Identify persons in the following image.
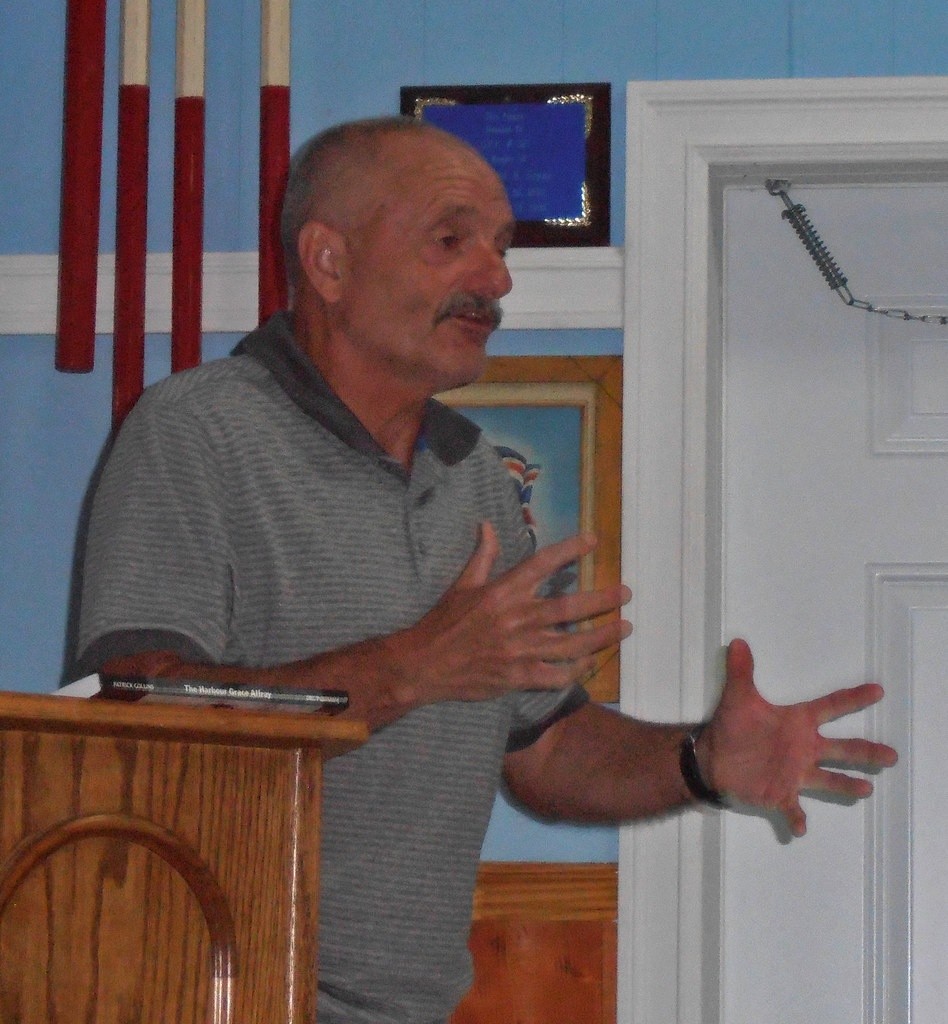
[71,115,900,1024]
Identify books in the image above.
[49,671,349,715]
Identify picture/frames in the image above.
[399,83,613,248]
[430,354,622,703]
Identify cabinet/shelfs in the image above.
[0,693,369,1024]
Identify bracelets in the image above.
[680,721,727,810]
